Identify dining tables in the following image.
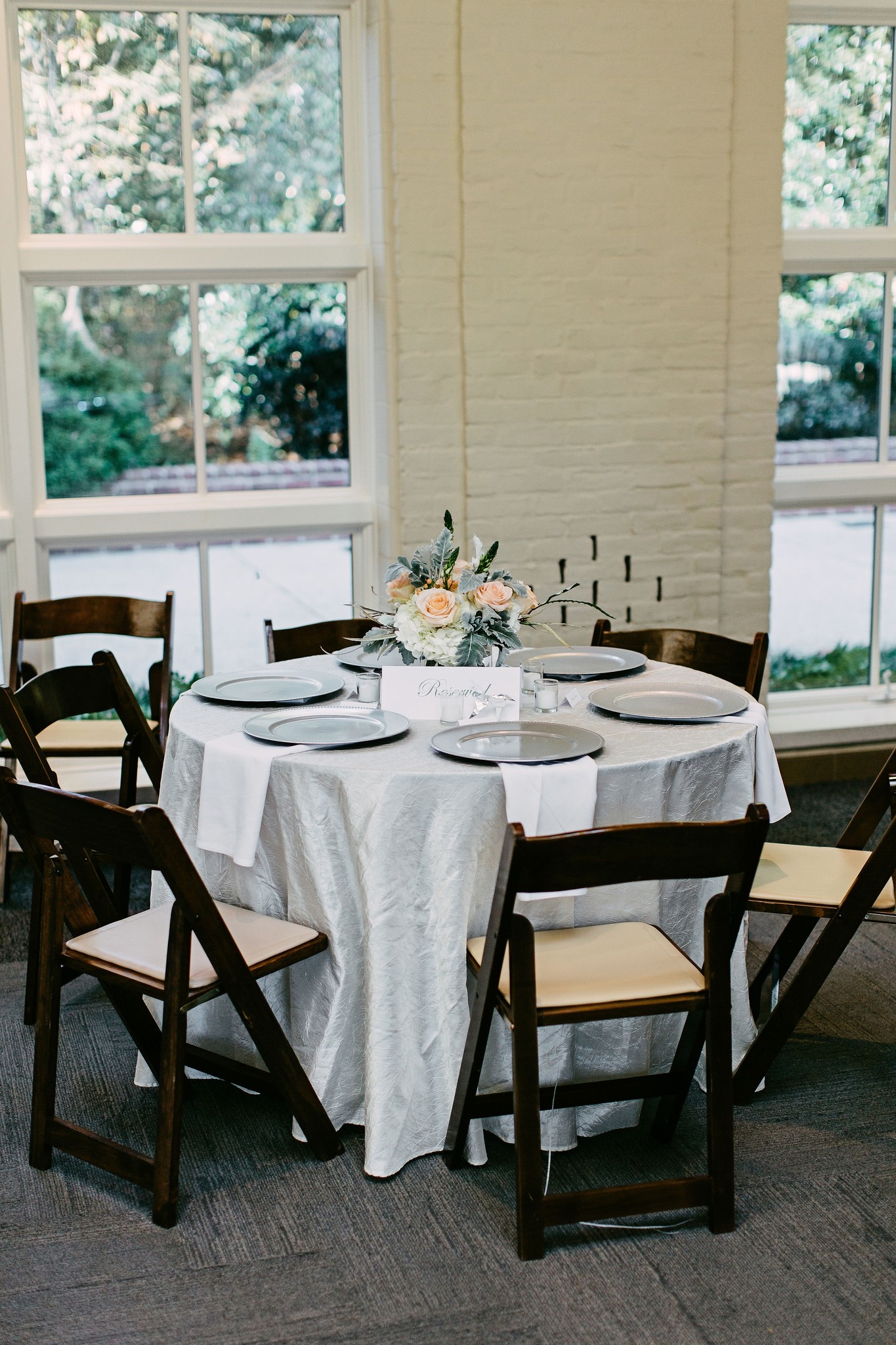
[134,644,790,1178]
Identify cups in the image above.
[356,671,381,704]
[534,678,559,712]
[519,661,544,694]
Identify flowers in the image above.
[320,509,613,669]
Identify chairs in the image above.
[590,619,768,700]
[0,762,348,1231]
[3,647,164,1023]
[261,617,378,664]
[5,588,172,911]
[439,802,770,1263]
[729,750,896,1106]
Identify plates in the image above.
[242,704,411,746]
[335,645,423,669]
[503,646,648,675]
[587,682,749,720]
[190,669,346,702]
[429,720,604,764]
